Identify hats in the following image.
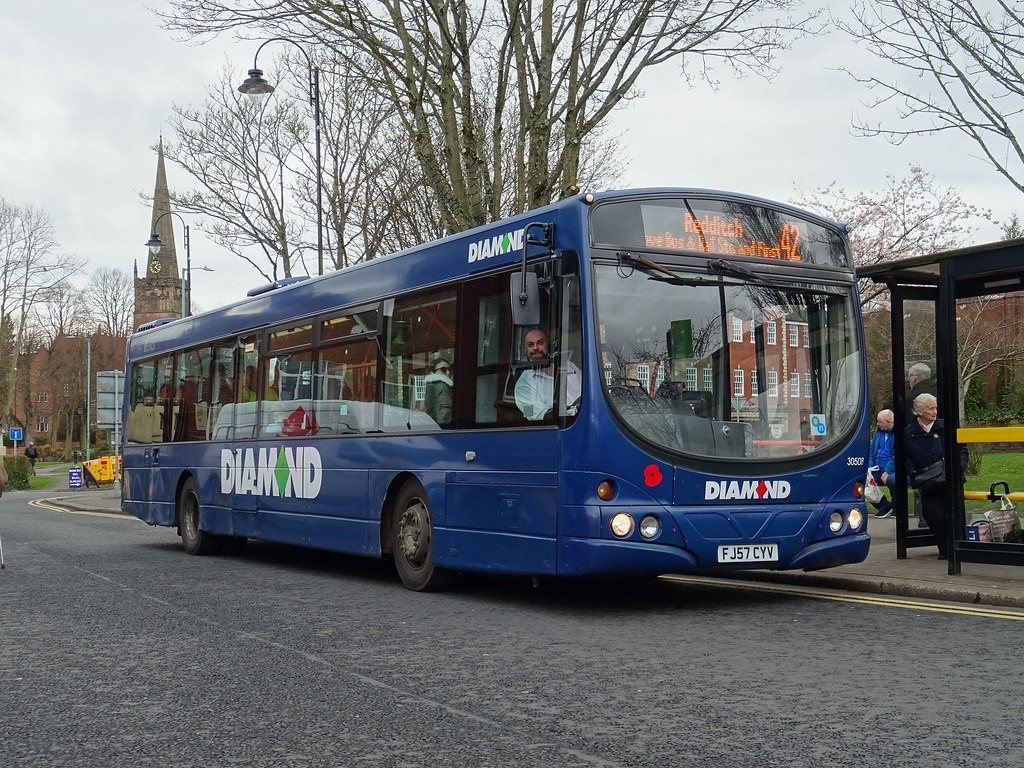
[29,442,33,446]
[208,362,229,376]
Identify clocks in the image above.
[149,261,162,274]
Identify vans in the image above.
[213,400,442,435]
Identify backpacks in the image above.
[28,447,35,457]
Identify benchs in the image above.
[963,481,1023,502]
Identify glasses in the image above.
[434,367,451,374]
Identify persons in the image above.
[514,329,582,420]
[867,409,909,518]
[906,362,939,527]
[906,393,969,560]
[202,361,233,406]
[424,358,453,425]
[354,373,390,404]
[242,366,278,403]
[159,384,170,397]
[24,441,38,476]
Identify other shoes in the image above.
[937,554,947,560]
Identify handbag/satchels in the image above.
[983,495,1021,542]
[913,461,945,492]
[864,465,883,504]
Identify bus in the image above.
[120,186,876,596]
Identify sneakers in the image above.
[891,512,896,518]
[873,502,893,518]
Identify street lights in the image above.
[141,212,193,316]
[179,264,216,391]
[238,35,325,275]
